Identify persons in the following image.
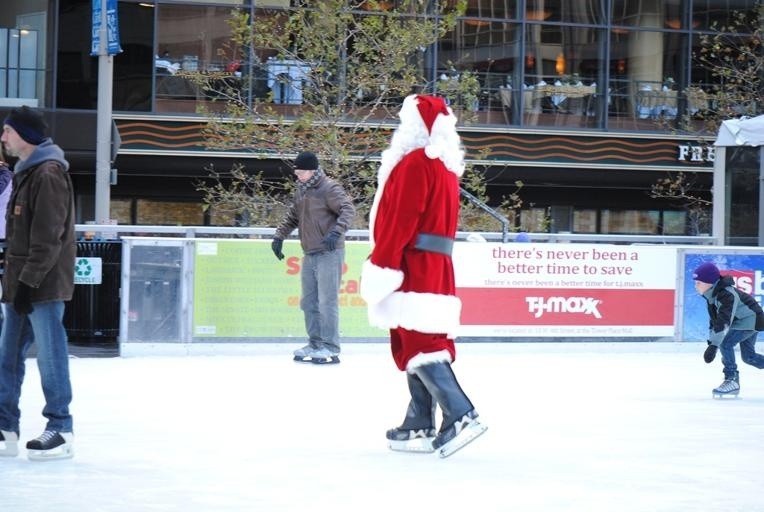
[0,104,75,460]
[357,94,487,457]
[691,262,763,399]
[0,144,16,338]
[272,152,354,364]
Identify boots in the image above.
[293,345,314,358]
[1,429,20,442]
[386,372,437,441]
[310,346,340,358]
[26,430,76,451]
[713,372,740,396]
[410,359,479,451]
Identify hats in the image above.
[692,264,721,283]
[293,151,319,173]
[3,106,49,146]
[399,94,462,158]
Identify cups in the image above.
[83,221,95,241]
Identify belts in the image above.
[411,231,455,259]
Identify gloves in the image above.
[270,238,287,262]
[320,232,342,254]
[13,282,36,313]
[704,344,718,364]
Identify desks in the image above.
[152,48,325,125]
[436,71,710,125]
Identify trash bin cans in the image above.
[62,238,124,342]
